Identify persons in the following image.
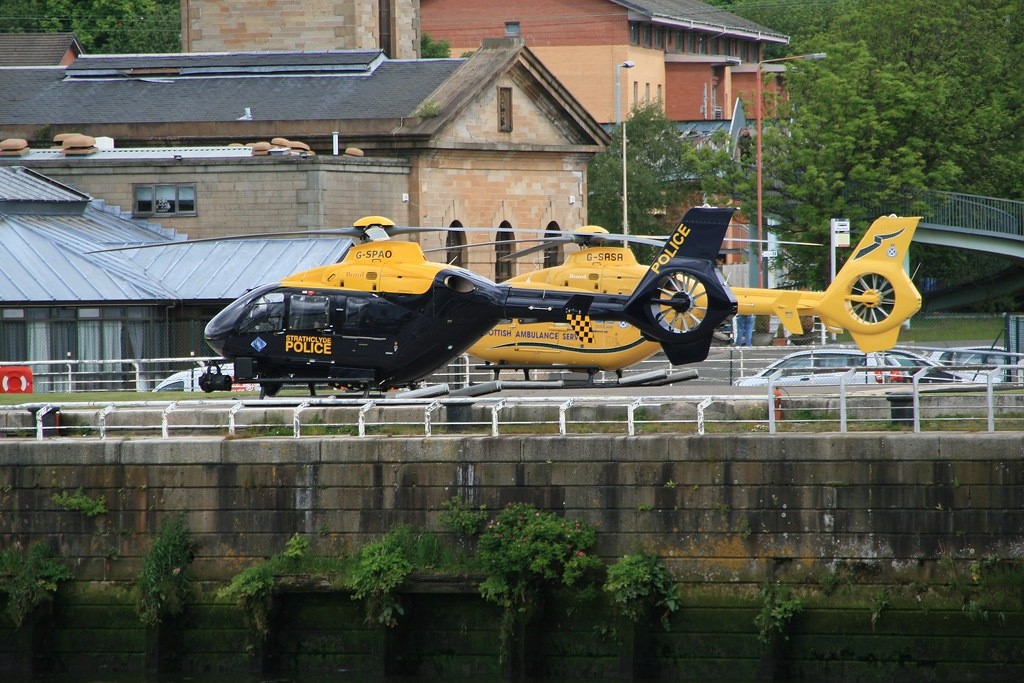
[736,314,756,346]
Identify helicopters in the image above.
[421,208,922,388]
[81,205,738,408]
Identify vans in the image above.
[152,362,262,394]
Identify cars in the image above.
[727,350,913,385]
[884,349,963,382]
[928,343,1007,383]
